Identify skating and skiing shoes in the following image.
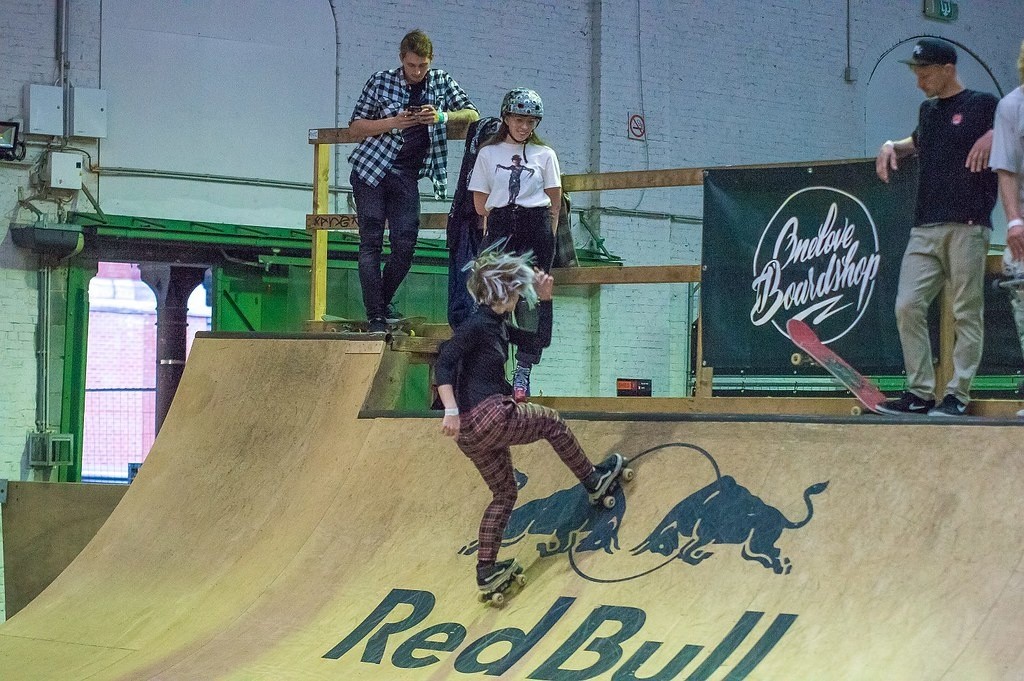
[477,557,525,604]
[583,453,634,509]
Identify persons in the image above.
[988,37,1023,264]
[348,27,480,335]
[874,40,1000,417]
[465,87,565,401]
[432,233,620,595]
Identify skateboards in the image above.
[784,317,888,416]
[320,314,428,337]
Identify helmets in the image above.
[501,87,544,121]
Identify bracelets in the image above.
[444,408,459,415]
[442,112,448,124]
[1006,220,1022,230]
[437,112,444,123]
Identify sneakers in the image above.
[369,303,388,334]
[875,391,934,415]
[378,301,404,322]
[926,395,968,417]
[510,365,531,398]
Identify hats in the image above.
[896,38,958,65]
